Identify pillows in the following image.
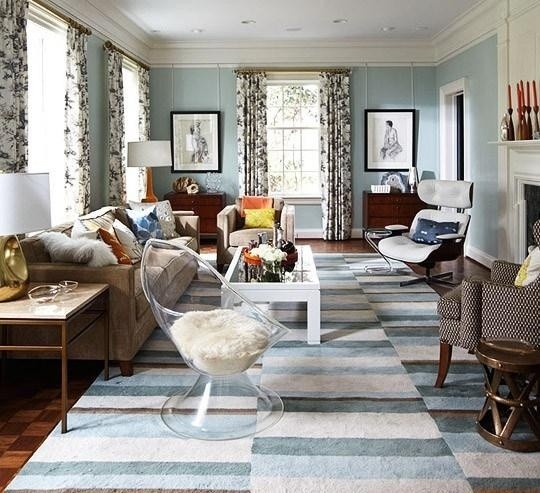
[242,208,276,229]
[514,244,539,288]
[40,206,179,267]
[412,216,460,244]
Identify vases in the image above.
[259,259,282,281]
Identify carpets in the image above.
[0,249,540,493]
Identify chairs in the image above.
[137,238,292,441]
[434,217,540,392]
[377,178,474,288]
[215,196,295,273]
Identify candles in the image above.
[505,79,540,112]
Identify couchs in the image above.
[1,207,200,377]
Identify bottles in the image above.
[500,111,508,140]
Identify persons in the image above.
[188,118,204,162]
[380,119,403,158]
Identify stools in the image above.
[471,331,539,451]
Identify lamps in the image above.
[127,139,172,202]
[0,171,52,301]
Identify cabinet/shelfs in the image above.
[362,189,437,240]
[163,190,226,240]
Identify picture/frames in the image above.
[362,107,416,172]
[170,110,222,173]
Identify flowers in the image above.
[248,242,289,265]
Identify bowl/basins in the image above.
[26,279,79,305]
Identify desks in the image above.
[361,224,410,274]
[0,279,110,434]
